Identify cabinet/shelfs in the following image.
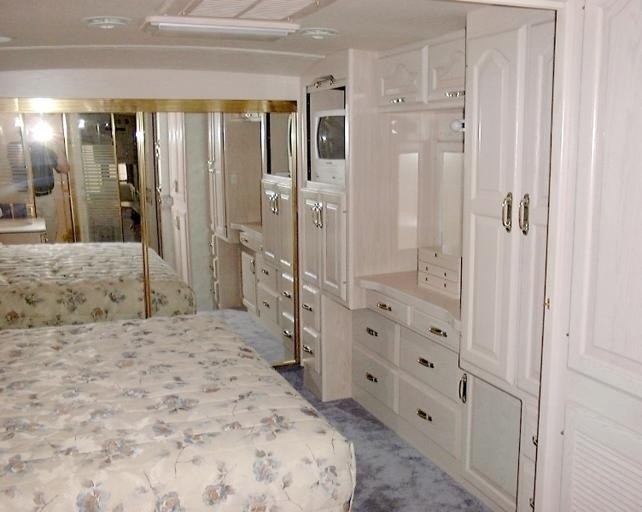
[297,0,556,512]
[158,111,295,356]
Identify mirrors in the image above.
[0,96,301,370]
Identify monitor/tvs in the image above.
[311,109,347,185]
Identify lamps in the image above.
[138,14,302,42]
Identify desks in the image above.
[0,216,47,245]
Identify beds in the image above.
[0,311,356,512]
[0,241,196,330]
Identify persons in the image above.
[7,116,69,244]
